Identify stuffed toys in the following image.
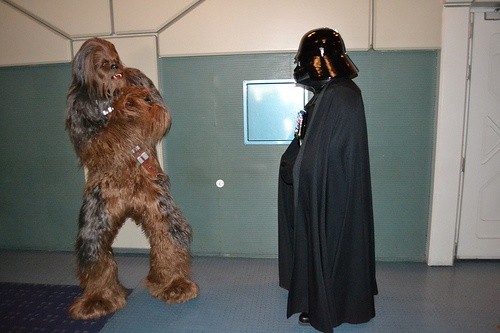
[68,37,199,321]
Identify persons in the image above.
[277,27,379,332]
